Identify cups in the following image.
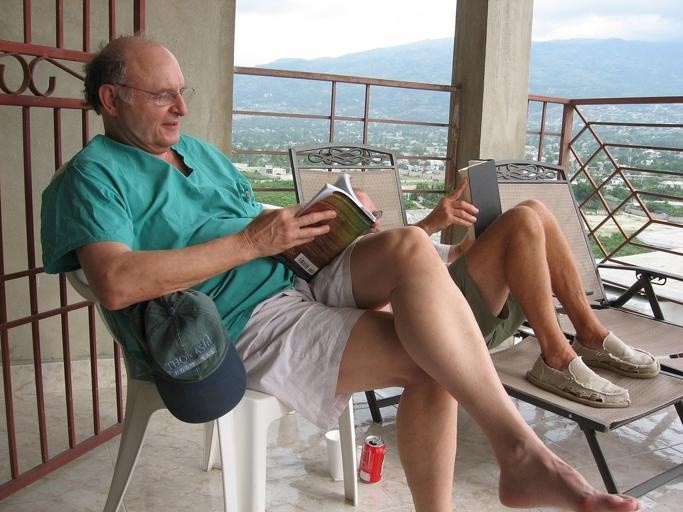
[325,428,344,484]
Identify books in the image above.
[458,159,502,241]
[269,174,376,283]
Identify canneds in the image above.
[358,436,386,484]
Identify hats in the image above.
[142,287,246,424]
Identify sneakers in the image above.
[571,332,661,378]
[526,350,631,408]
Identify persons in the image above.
[43,36,641,512]
[352,187,660,408]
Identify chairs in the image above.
[287,145,683,510]
[65,269,362,511]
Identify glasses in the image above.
[372,208,383,219]
[114,82,195,108]
